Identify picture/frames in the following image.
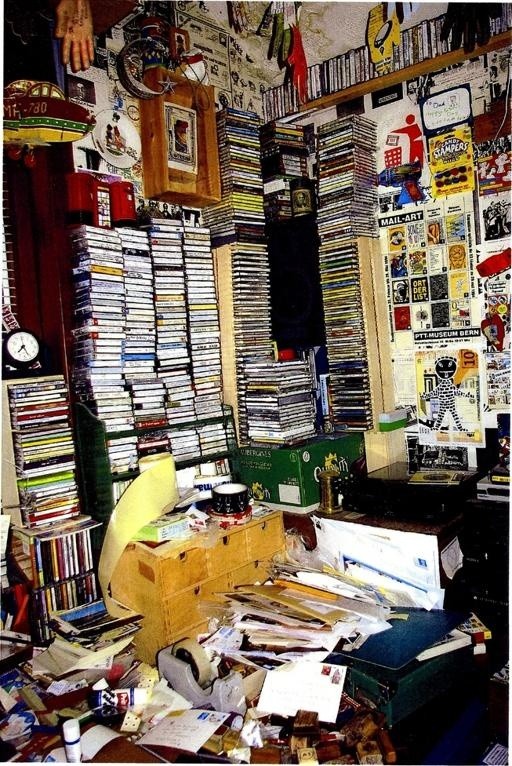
[170,26,191,61]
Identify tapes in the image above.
[172,638,211,686]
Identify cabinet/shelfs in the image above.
[110,508,286,669]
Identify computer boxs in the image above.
[365,461,491,523]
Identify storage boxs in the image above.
[238,433,370,504]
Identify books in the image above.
[2,3,510,697]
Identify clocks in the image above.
[4,328,43,370]
